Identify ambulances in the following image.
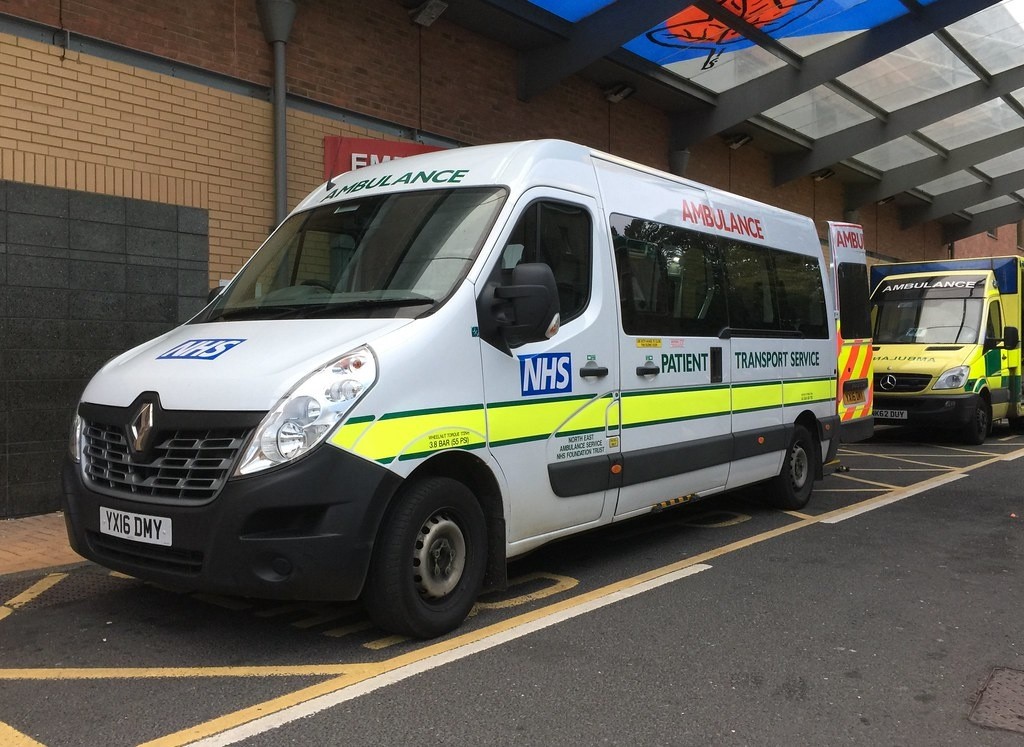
[62,133,877,640]
[866,253,1024,447]
